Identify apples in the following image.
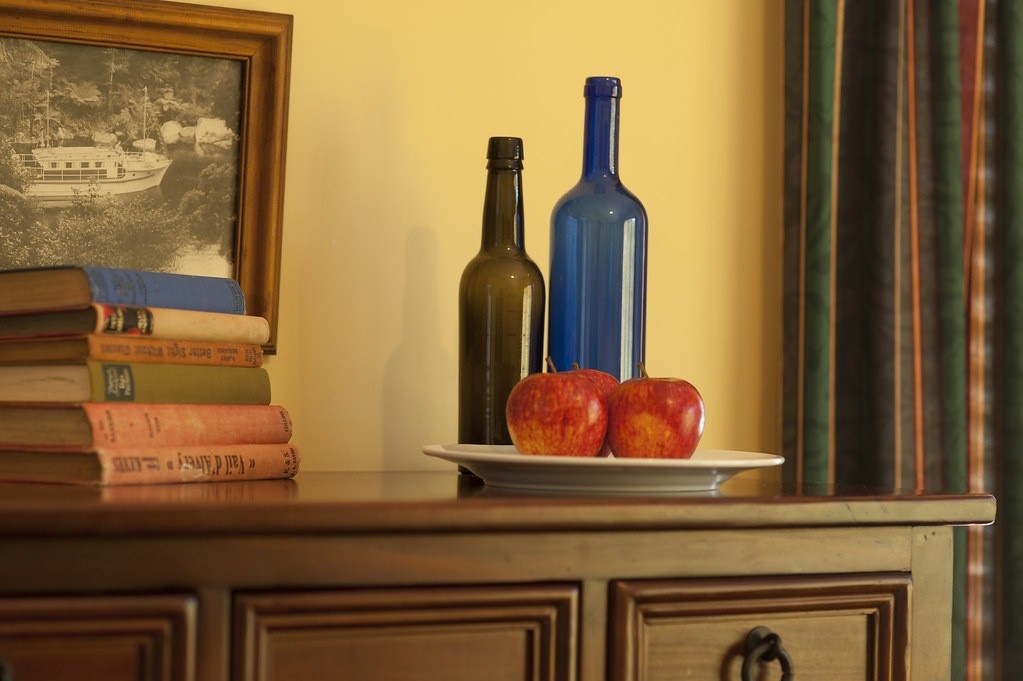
[507,356,705,461]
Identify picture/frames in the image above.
[2,2,295,359]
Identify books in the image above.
[0,266,301,483]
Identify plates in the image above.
[425,439,787,499]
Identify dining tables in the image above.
[2,470,997,679]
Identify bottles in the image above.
[457,135,543,475]
[545,74,648,381]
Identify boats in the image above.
[0,41,177,207]
